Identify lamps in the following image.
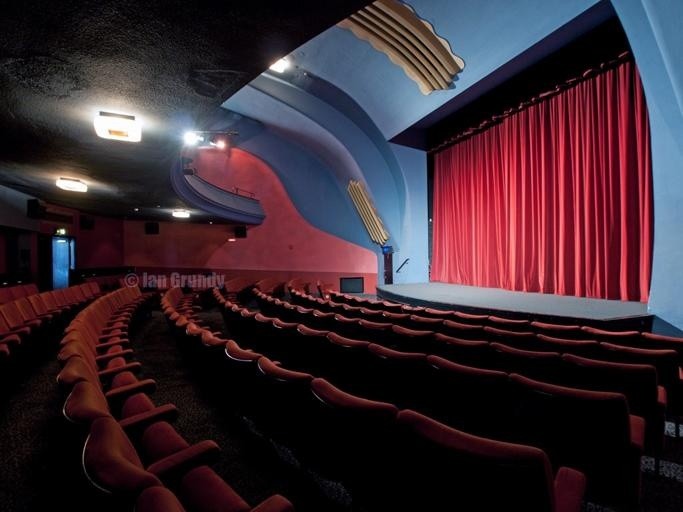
[172,211,190,220]
[54,177,88,193]
[92,111,142,143]
